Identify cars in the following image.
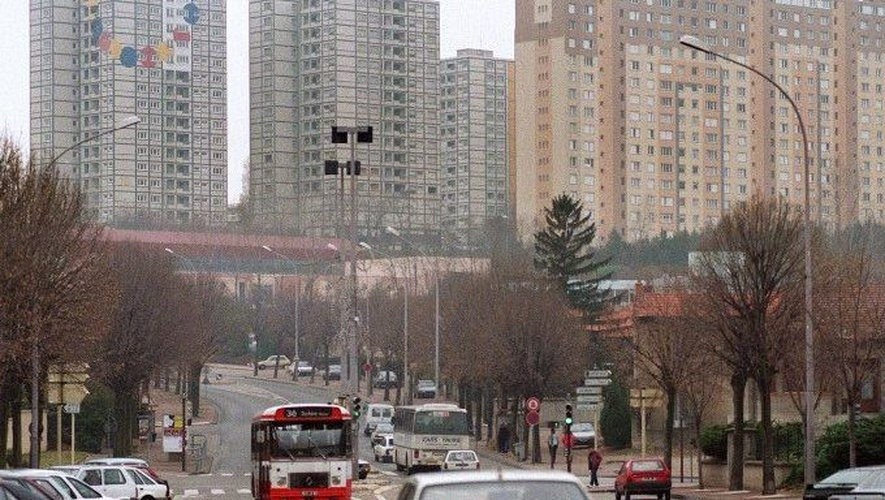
[368,423,395,462]
[415,379,437,399]
[800,462,884,500]
[396,466,592,500]
[285,360,317,375]
[0,457,169,500]
[322,365,340,380]
[441,449,481,472]
[357,460,371,479]
[374,371,398,388]
[567,424,598,447]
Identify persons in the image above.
[497,421,511,453]
[548,428,558,464]
[563,427,574,464]
[273,424,342,458]
[587,445,603,488]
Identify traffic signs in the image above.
[575,370,611,411]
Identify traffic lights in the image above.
[566,403,572,424]
[352,396,361,418]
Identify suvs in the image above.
[613,457,674,500]
[258,354,292,371]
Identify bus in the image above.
[249,402,354,500]
[389,403,470,476]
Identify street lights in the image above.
[359,241,410,407]
[263,245,299,381]
[29,116,142,471]
[679,34,819,490]
[165,248,198,284]
[384,225,440,404]
[328,242,373,396]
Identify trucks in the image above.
[364,404,395,434]
